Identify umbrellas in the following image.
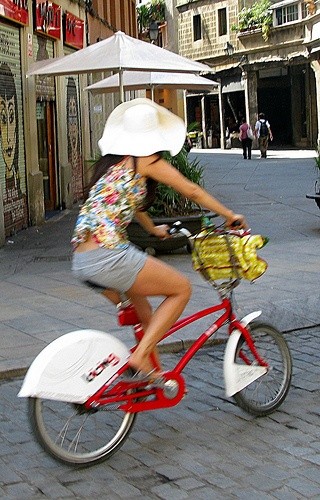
[85,70,220,102]
[29,31,214,102]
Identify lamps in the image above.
[224,42,234,58]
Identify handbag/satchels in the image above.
[246,124,256,141]
[189,229,271,286]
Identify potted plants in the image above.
[84,121,220,251]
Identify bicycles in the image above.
[14,218,294,470]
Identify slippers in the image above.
[124,365,165,382]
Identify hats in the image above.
[98,97,187,156]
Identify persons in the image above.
[253,113,273,159]
[239,118,253,160]
[72,97,251,397]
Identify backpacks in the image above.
[258,120,269,138]
